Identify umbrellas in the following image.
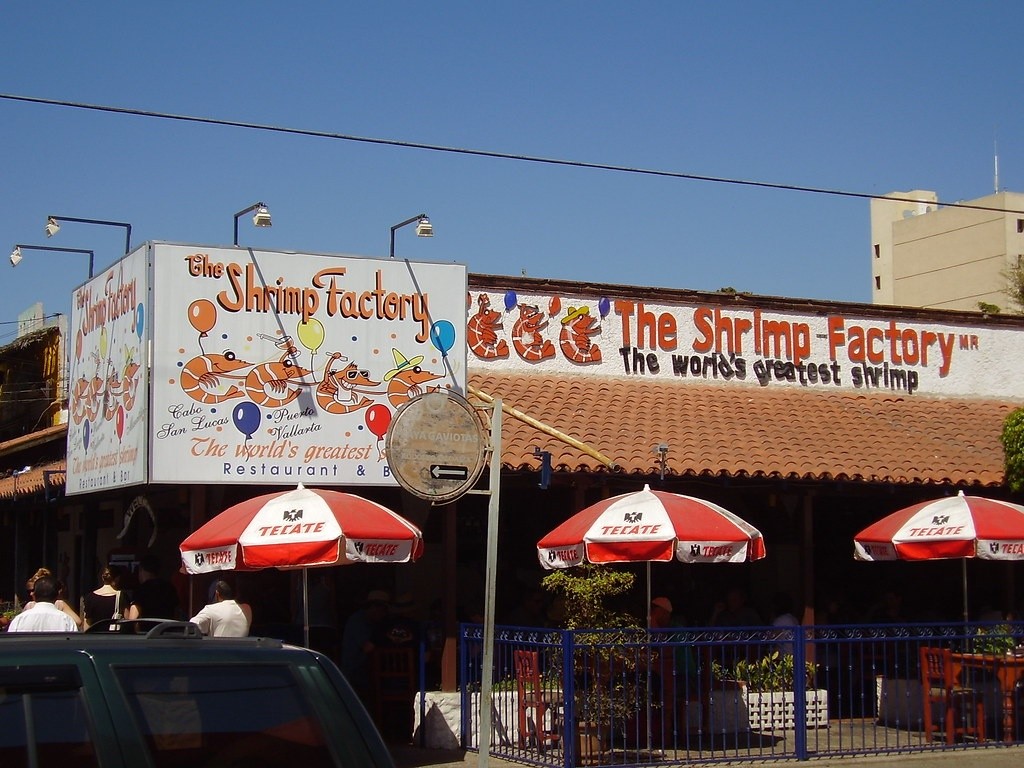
[852,488,1024,651]
[178,481,427,650]
[536,483,769,631]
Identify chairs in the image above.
[672,642,711,749]
[920,648,984,745]
[514,649,565,752]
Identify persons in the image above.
[764,591,801,660]
[127,556,179,633]
[188,578,253,637]
[347,589,549,702]
[7,576,80,633]
[83,562,141,633]
[707,586,767,659]
[649,594,701,739]
[20,567,83,628]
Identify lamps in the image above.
[233,200,273,247]
[8,243,95,279]
[46,215,133,256]
[389,214,434,259]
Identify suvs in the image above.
[1,615,401,768]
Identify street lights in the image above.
[10,244,95,280]
[46,214,132,255]
[389,212,434,260]
[232,201,272,248]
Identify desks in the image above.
[949,653,1024,746]
[589,644,675,749]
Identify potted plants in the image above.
[541,561,657,768]
